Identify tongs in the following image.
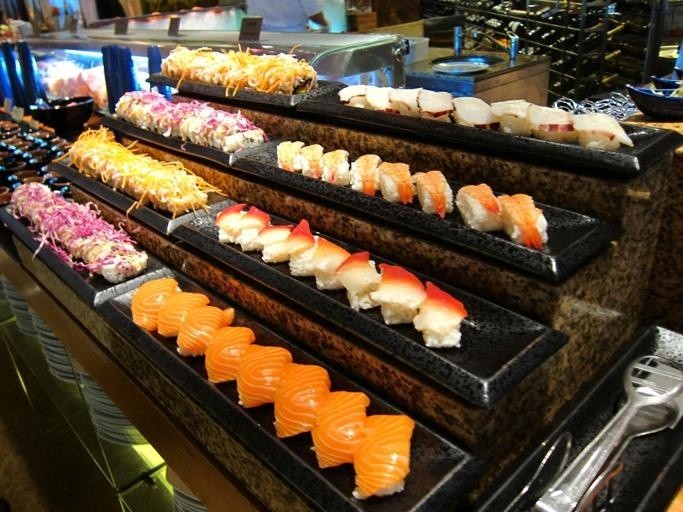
[534,355,682,512]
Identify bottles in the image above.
[532,0,627,101]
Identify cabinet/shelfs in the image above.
[613,0,664,82]
[452,0,610,104]
[0,70,682,511]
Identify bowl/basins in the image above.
[29,97,92,135]
[625,83,683,121]
[0,135,71,203]
[650,75,683,88]
[1,107,28,135]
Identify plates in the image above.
[430,62,488,76]
